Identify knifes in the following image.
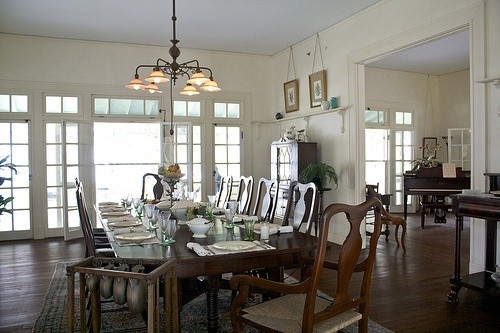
[252,242,267,250]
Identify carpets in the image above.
[33,261,395,333]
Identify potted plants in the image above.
[303,161,337,189]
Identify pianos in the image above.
[404,161,471,221]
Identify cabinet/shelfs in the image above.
[269,141,317,219]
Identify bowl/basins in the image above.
[186,215,213,238]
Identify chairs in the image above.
[74,176,317,326]
[232,197,383,331]
[365,182,407,253]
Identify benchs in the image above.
[420,201,453,229]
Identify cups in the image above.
[225,209,234,228]
[228,202,239,218]
[243,219,255,241]
[260,221,270,243]
[206,195,216,218]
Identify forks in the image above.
[203,244,215,254]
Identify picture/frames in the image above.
[307,70,329,108]
[422,136,437,159]
[283,79,300,113]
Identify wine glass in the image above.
[127,197,176,246]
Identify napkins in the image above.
[101,203,293,257]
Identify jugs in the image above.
[294,129,305,142]
[281,126,296,142]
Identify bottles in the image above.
[275,112,282,120]
[321,101,330,110]
[330,97,336,109]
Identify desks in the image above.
[366,194,393,242]
[92,201,331,333]
[446,194,500,307]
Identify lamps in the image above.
[125,0,221,96]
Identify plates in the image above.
[213,240,256,251]
[101,211,130,216]
[220,214,248,219]
[254,224,282,230]
[115,232,156,241]
[109,221,143,227]
[98,205,122,208]
